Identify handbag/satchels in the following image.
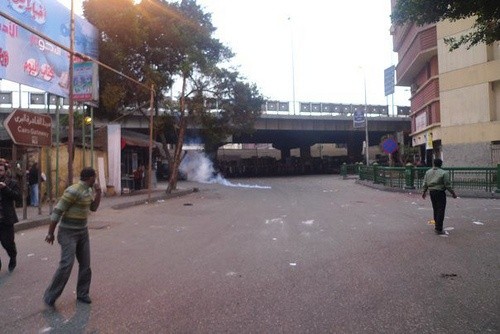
[40,172,46,181]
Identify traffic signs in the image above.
[3,108,53,147]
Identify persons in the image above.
[43,167,101,308]
[421,157,458,235]
[0,164,21,273]
[28,162,46,208]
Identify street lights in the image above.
[358,66,369,168]
[288,16,296,115]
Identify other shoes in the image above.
[8,255,16,272]
[435,230,445,235]
[77,295,92,304]
